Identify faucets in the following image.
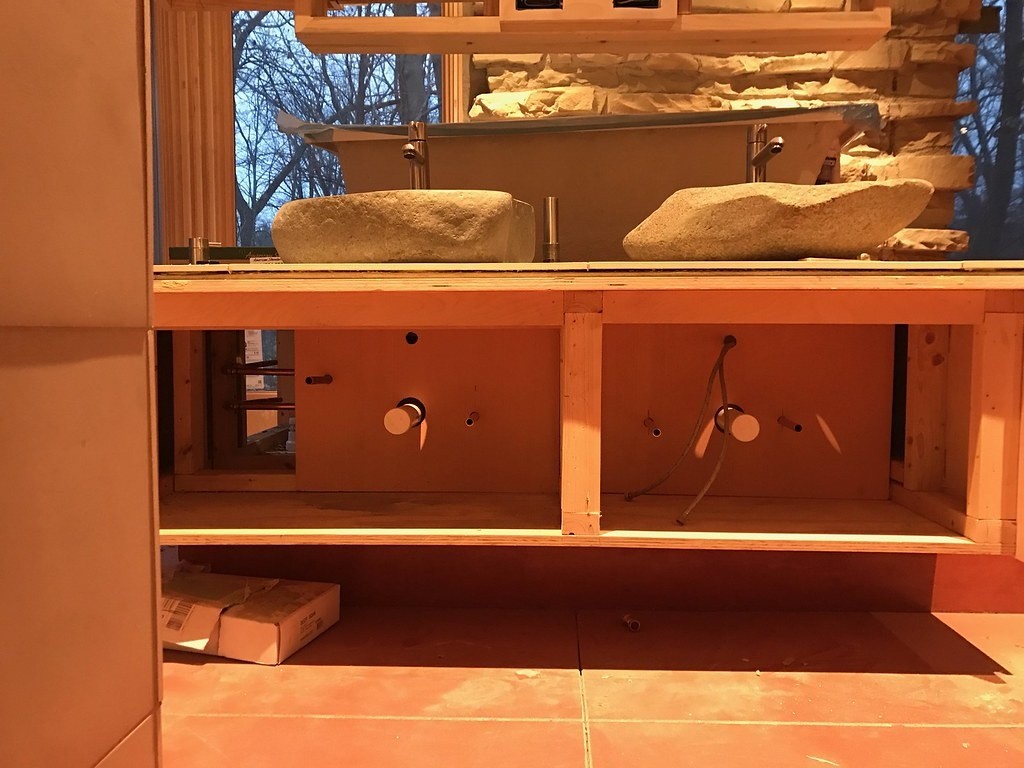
[401,119,430,190]
[746,123,785,183]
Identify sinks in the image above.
[623,176,936,260]
[271,189,536,264]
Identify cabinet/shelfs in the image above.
[150,259,1024,555]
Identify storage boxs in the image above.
[156,571,343,666]
